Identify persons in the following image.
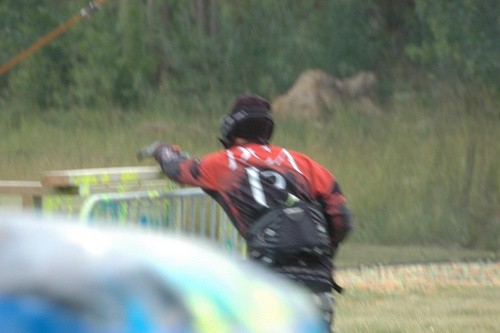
[136,94,351,333]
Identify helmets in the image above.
[218,95,274,145]
